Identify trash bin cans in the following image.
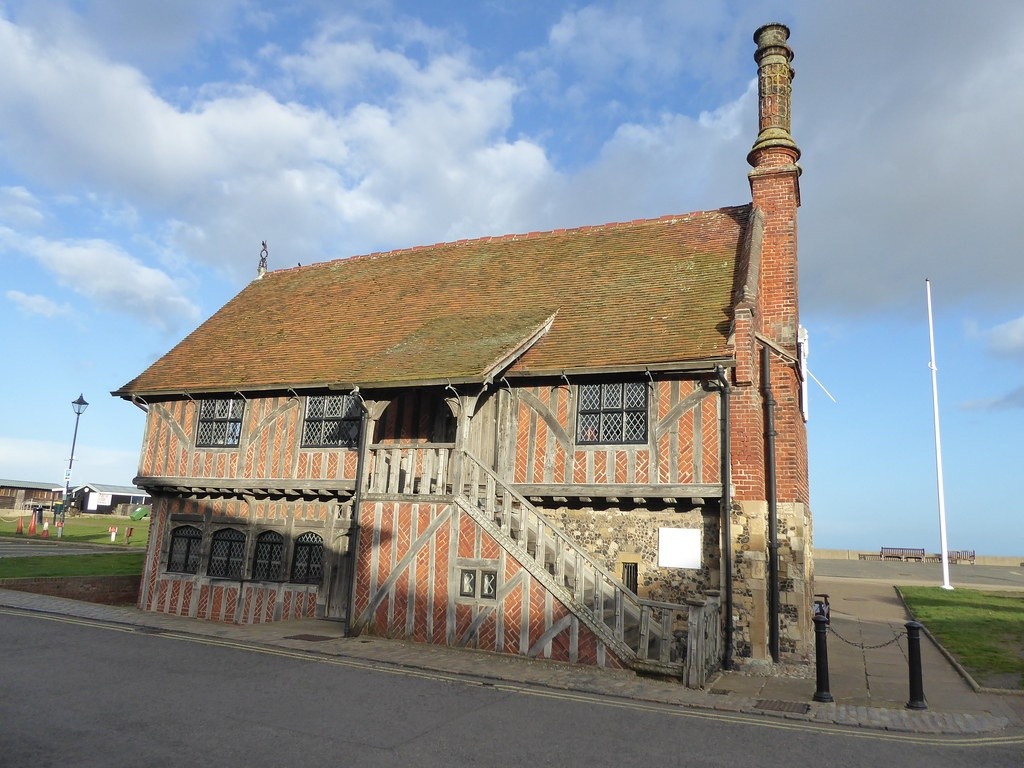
[54,503,63,514]
[33,507,43,525]
[130,507,149,521]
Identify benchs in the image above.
[879,546,976,564]
[814,594,830,626]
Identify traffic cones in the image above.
[26,508,36,536]
[15,516,23,535]
[41,517,49,538]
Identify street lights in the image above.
[59,392,90,524]
